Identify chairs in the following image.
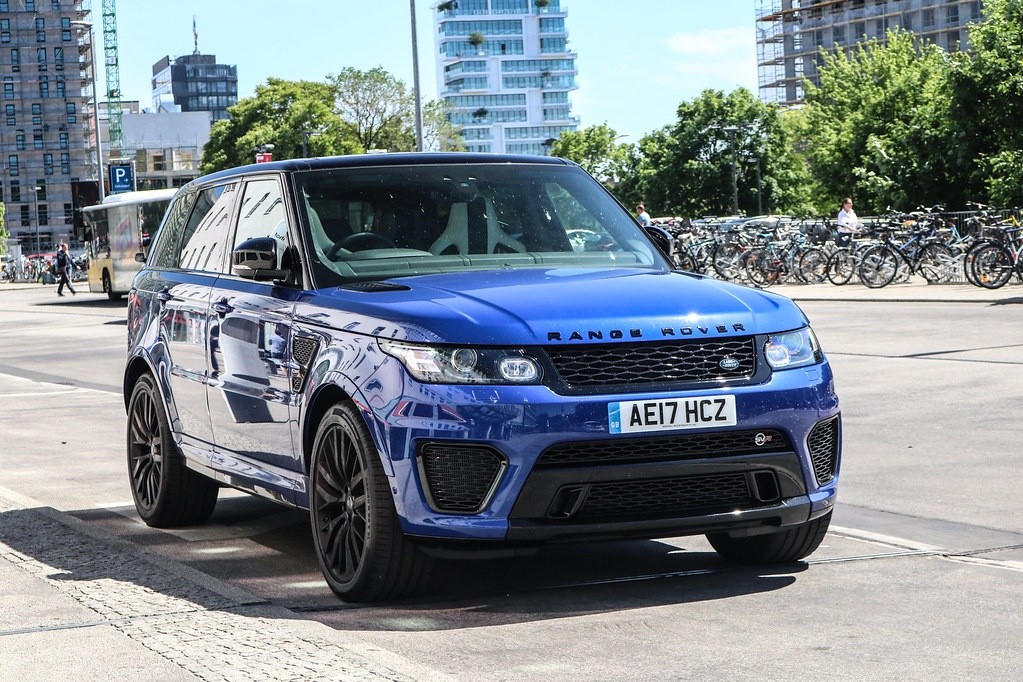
[428,197,528,255]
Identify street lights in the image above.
[67,20,105,201]
[28,184,42,273]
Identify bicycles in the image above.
[0,257,47,284]
[641,200,1023,289]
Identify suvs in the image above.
[121,150,843,605]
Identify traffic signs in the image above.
[111,165,133,194]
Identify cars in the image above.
[584,231,623,252]
[563,230,600,253]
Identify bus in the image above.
[76,188,189,299]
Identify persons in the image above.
[503,425,512,441]
[268,330,289,379]
[834,198,859,272]
[57,243,81,296]
[204,297,235,377]
[635,205,650,226]
